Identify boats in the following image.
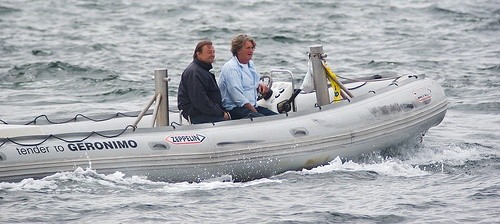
[0,44,448,186]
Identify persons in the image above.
[216,34,279,119]
[177,41,229,123]
